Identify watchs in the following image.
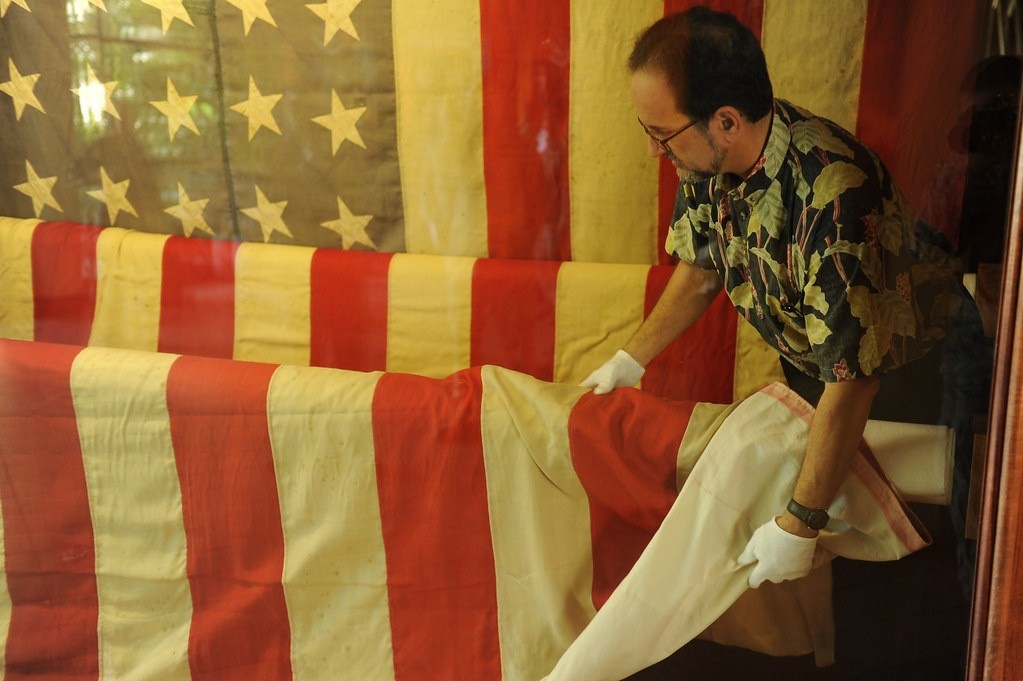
[787,499,830,530]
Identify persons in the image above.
[580,5,990,591]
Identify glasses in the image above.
[637,106,743,153]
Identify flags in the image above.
[2,1,982,267]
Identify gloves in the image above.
[737,515,820,589]
[577,350,645,395]
